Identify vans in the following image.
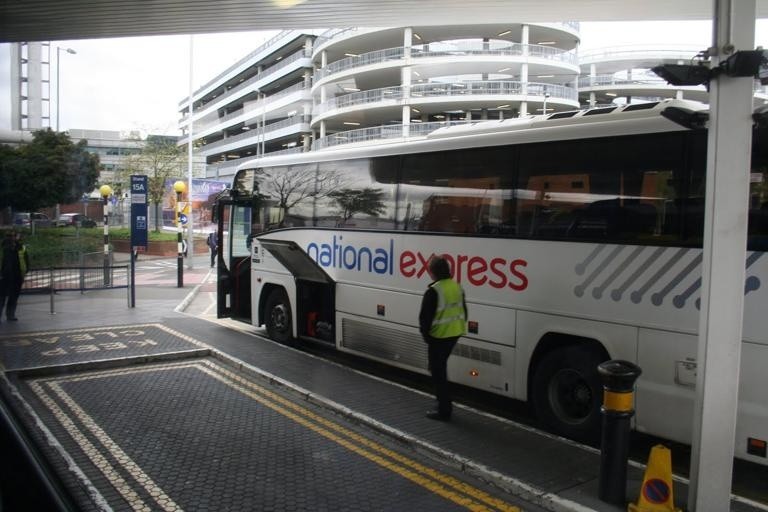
[13,212,52,229]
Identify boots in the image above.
[426,395,456,422]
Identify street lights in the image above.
[56,46,76,134]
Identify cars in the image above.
[53,213,96,229]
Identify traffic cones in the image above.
[627,443,683,511]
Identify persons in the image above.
[1,232,29,322]
[417,256,468,418]
[209,227,218,267]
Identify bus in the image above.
[206,97,766,469]
